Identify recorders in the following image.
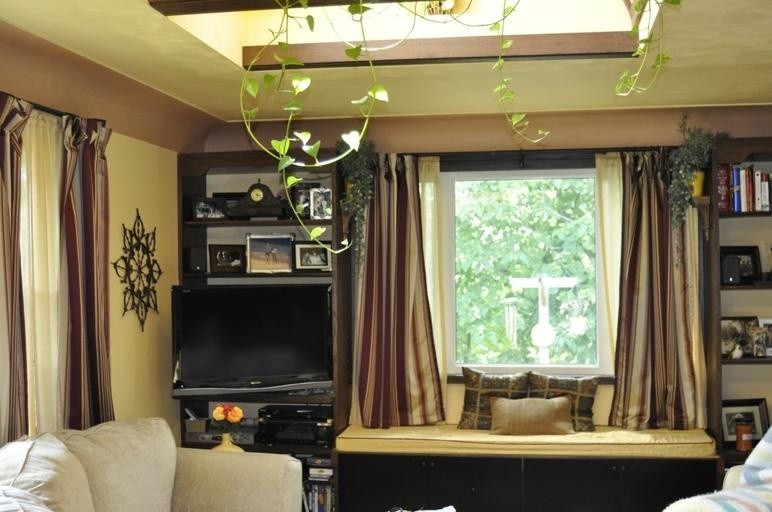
[258,405,333,448]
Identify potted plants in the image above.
[336,137,379,279]
[661,108,730,270]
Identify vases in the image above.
[212,430,244,453]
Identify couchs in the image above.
[0,411,304,511]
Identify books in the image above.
[716,163,770,214]
[292,455,336,511]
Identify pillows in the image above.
[527,371,602,433]
[487,394,575,437]
[455,364,532,432]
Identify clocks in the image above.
[226,174,287,220]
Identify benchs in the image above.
[330,418,724,511]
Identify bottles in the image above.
[736,417,753,452]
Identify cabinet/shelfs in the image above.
[700,131,772,476]
[175,145,351,511]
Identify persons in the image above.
[216,244,325,270]
[296,190,331,220]
[721,316,772,357]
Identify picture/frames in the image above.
[307,186,332,222]
[243,232,295,276]
[206,243,245,275]
[757,319,772,357]
[720,243,764,280]
[719,314,759,361]
[291,239,332,274]
[719,396,770,449]
[291,181,321,222]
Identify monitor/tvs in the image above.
[168,282,333,398]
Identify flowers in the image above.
[209,403,245,432]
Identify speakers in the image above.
[188,245,205,273]
[721,256,742,285]
